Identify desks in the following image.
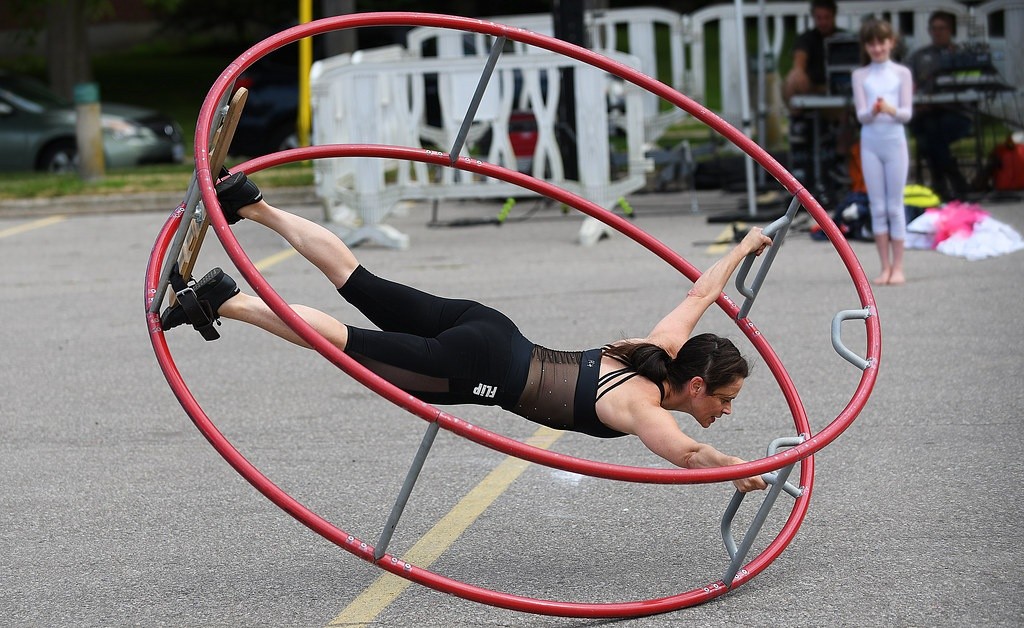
[791,89,984,214]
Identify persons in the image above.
[905,9,997,201]
[851,18,915,286]
[159,170,775,492]
[782,0,844,118]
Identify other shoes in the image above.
[160,268,236,330]
[210,170,263,228]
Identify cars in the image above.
[0,67,189,176]
